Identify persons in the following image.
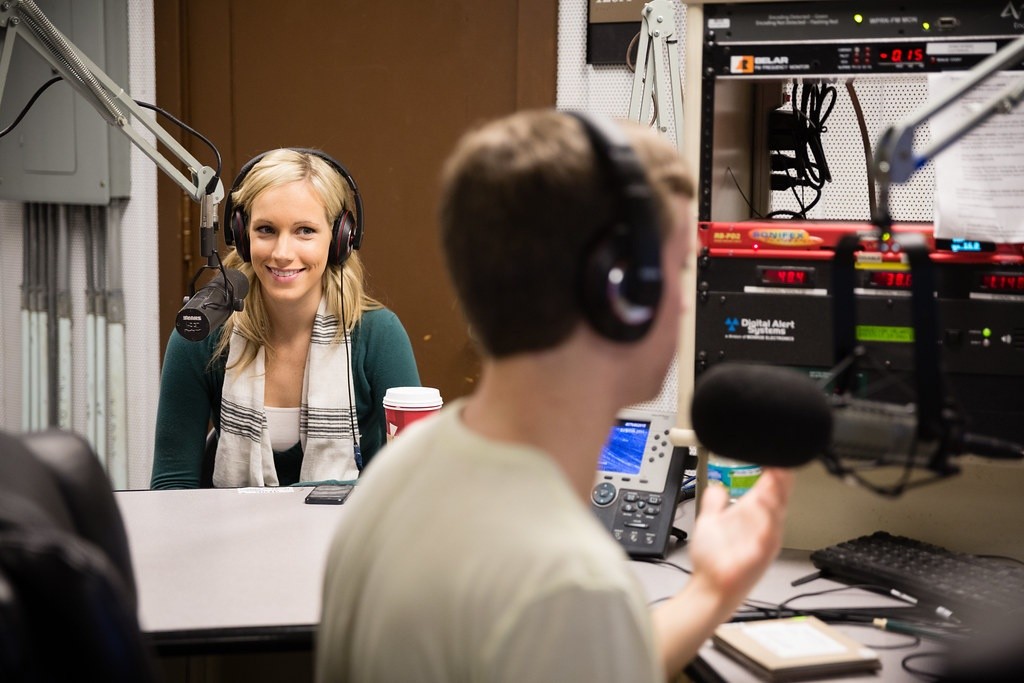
[314,107,796,683]
[150,148,424,490]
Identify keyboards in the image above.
[809,531,1023,624]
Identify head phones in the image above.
[224,148,364,265]
[563,107,669,345]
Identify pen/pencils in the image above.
[874,616,963,644]
[790,571,822,586]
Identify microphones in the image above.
[691,365,940,467]
[175,268,249,342]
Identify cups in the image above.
[382,387,443,448]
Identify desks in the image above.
[110,482,949,682]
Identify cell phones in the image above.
[305,484,354,505]
